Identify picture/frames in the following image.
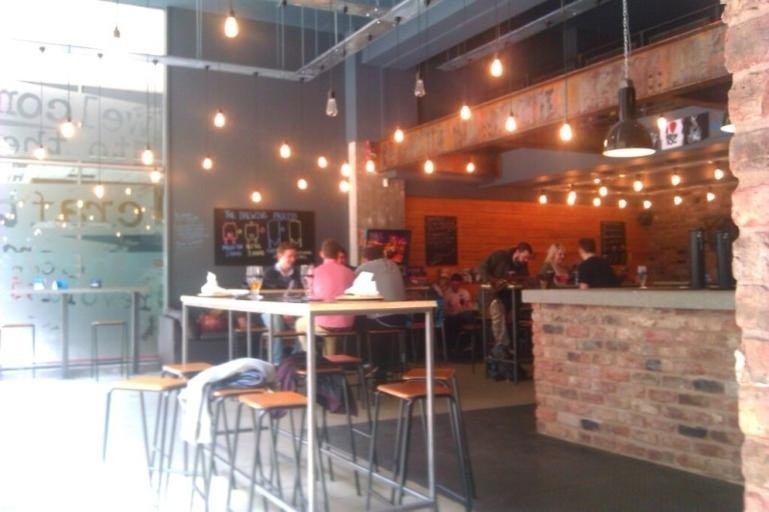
[364,228,410,278]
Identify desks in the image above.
[11,287,151,361]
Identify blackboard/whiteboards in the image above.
[425,216,457,265]
[214,207,316,266]
[601,222,627,265]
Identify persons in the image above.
[261,237,410,376]
[538,243,576,289]
[577,238,616,289]
[476,237,532,361]
[442,273,473,311]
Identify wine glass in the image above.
[244,265,266,299]
[298,264,318,302]
[638,265,647,289]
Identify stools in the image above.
[2,324,38,367]
[91,319,131,381]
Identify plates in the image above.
[334,293,384,302]
[196,291,231,297]
